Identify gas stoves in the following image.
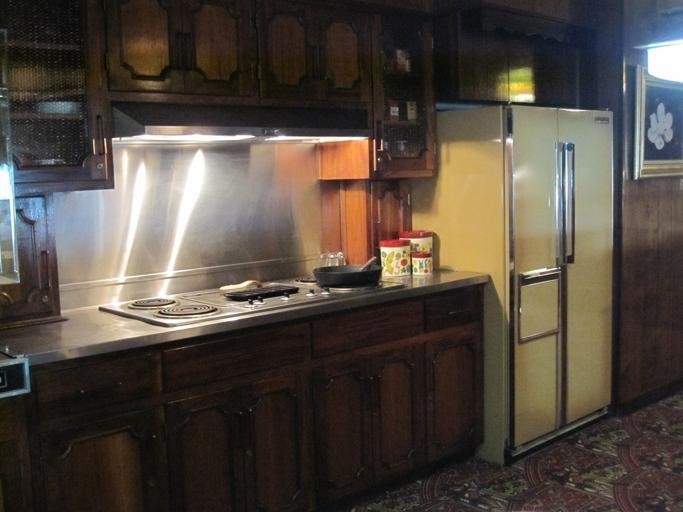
[98,281,406,326]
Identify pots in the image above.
[316,264,382,288]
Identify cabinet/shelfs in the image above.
[154,325,314,509]
[105,0,370,111]
[0,1,113,330]
[319,0,437,265]
[0,352,167,510]
[299,284,486,504]
[436,6,582,108]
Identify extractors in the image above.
[112,99,366,149]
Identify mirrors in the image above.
[632,36,683,181]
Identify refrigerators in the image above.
[411,105,616,471]
